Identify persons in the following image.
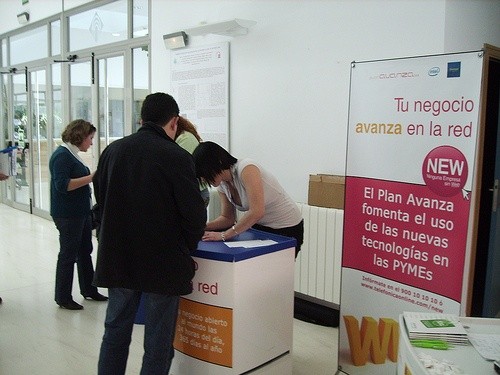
[92,92,207,375]
[191,141,304,259]
[173,116,210,208]
[49,119,109,310]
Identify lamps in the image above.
[17,12,29,24]
[163,31,188,49]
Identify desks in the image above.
[397,313,500,375]
[172,228,297,375]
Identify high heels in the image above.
[80,292,109,299]
[56,299,83,310]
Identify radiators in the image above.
[292,202,345,310]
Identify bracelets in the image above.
[232,226,240,235]
[221,232,226,241]
